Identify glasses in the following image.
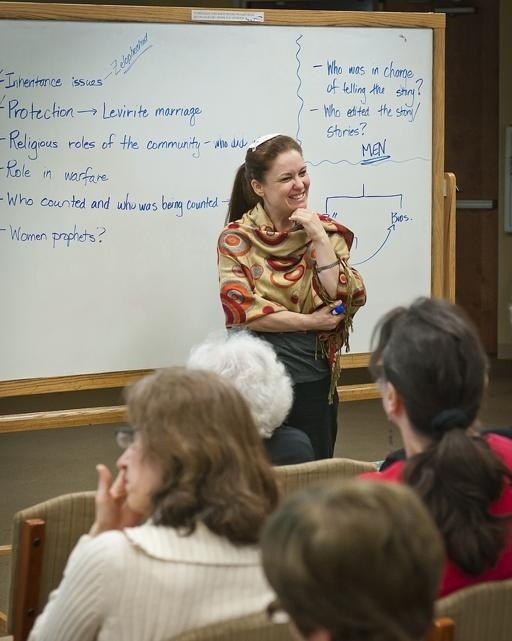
[268,597,292,625]
[112,426,141,451]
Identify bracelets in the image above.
[316,261,340,274]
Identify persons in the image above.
[355,292,512,604]
[255,476,445,640]
[26,362,281,640]
[185,330,319,469]
[216,130,370,461]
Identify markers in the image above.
[331,303,346,315]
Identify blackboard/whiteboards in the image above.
[0,0,448,397]
